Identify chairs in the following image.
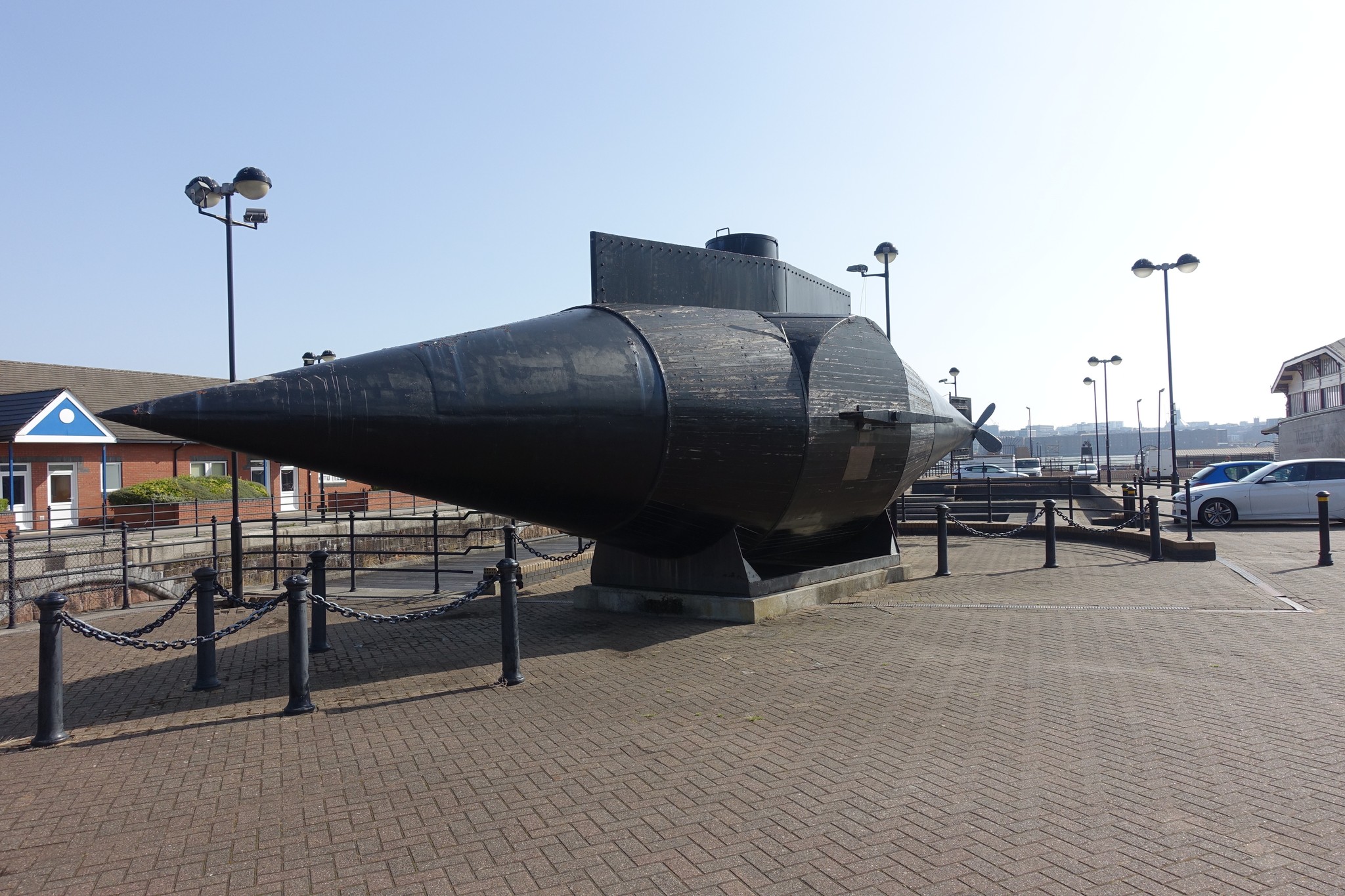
[1240,468,1248,479]
[1295,468,1306,481]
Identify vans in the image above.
[1143,448,1178,482]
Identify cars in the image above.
[1183,461,1306,493]
[951,464,1029,477]
[1075,463,1099,482]
[1173,458,1345,523]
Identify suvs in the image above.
[1014,458,1044,476]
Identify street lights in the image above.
[1083,377,1100,482]
[1157,388,1166,489]
[938,368,962,485]
[1031,439,1033,455]
[300,349,338,524]
[1036,442,1039,458]
[1026,407,1033,457]
[847,242,900,539]
[184,164,276,621]
[1040,444,1042,457]
[1137,399,1144,480]
[1087,355,1123,489]
[1130,254,1201,496]
